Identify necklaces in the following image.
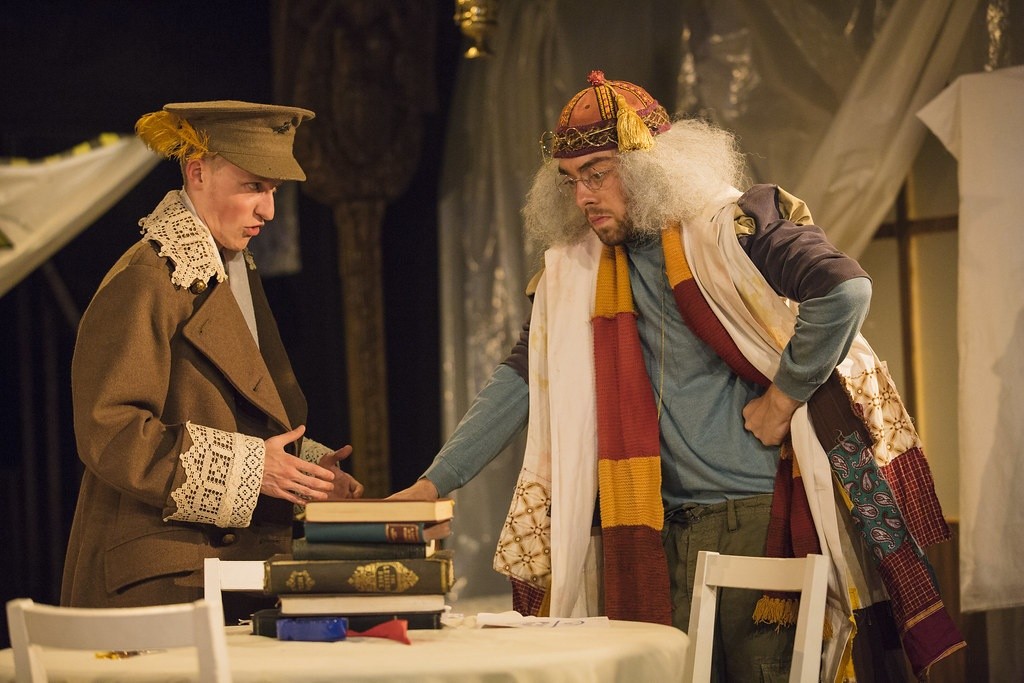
[651,262,666,420]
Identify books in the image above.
[262,498,456,612]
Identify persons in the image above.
[378,70,969,683]
[59,99,363,629]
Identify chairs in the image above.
[5,598,231,683]
[688,551,830,683]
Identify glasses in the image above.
[540,125,614,155]
[555,167,616,196]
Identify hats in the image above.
[163,99,316,181]
[553,70,670,158]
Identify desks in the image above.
[0,615,690,683]
